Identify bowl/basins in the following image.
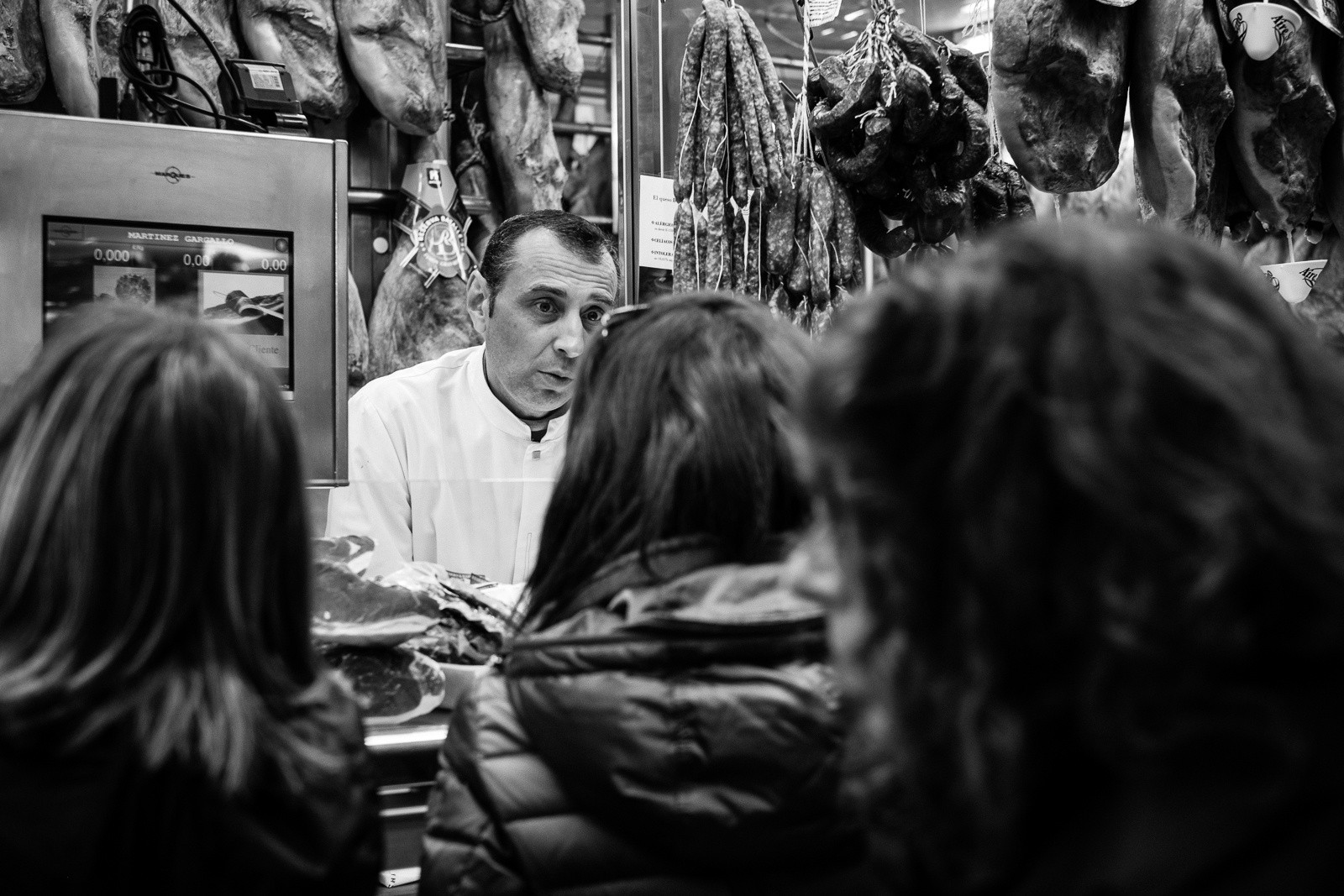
[436,662,489,709]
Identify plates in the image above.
[362,650,448,726]
[309,619,439,645]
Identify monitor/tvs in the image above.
[0,110,349,487]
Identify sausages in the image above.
[671,0,1036,343]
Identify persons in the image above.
[323,209,621,583]
[0,305,387,896]
[414,290,903,896]
[805,214,1344,896]
